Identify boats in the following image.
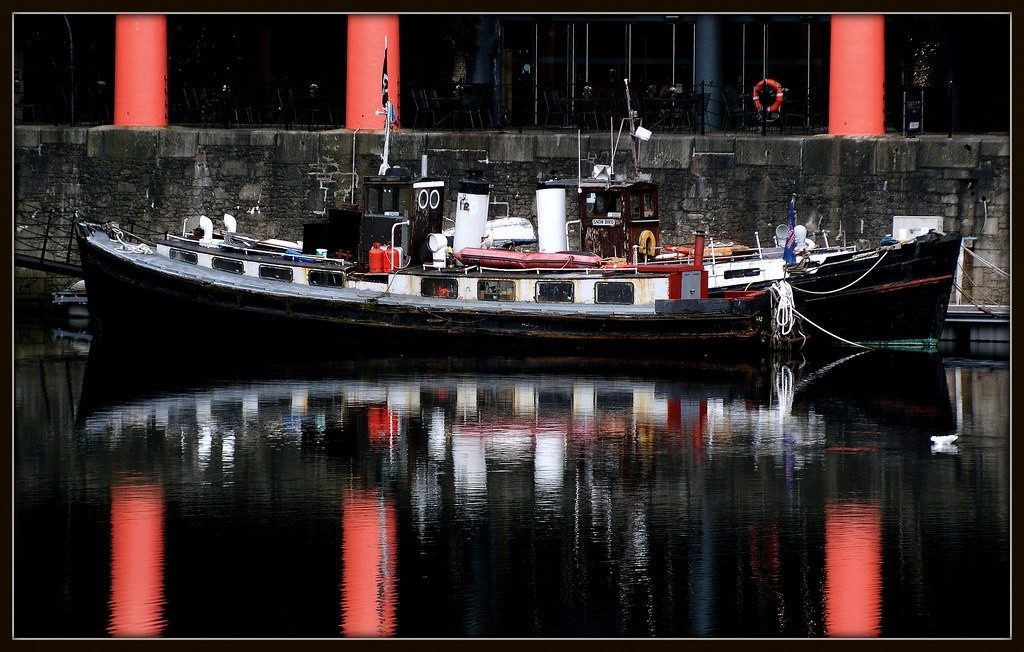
[74,327,961,456]
[71,101,963,348]
[52,273,87,310]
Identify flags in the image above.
[381,42,400,131]
[783,198,796,265]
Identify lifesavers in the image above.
[753,79,784,112]
[639,229,656,257]
